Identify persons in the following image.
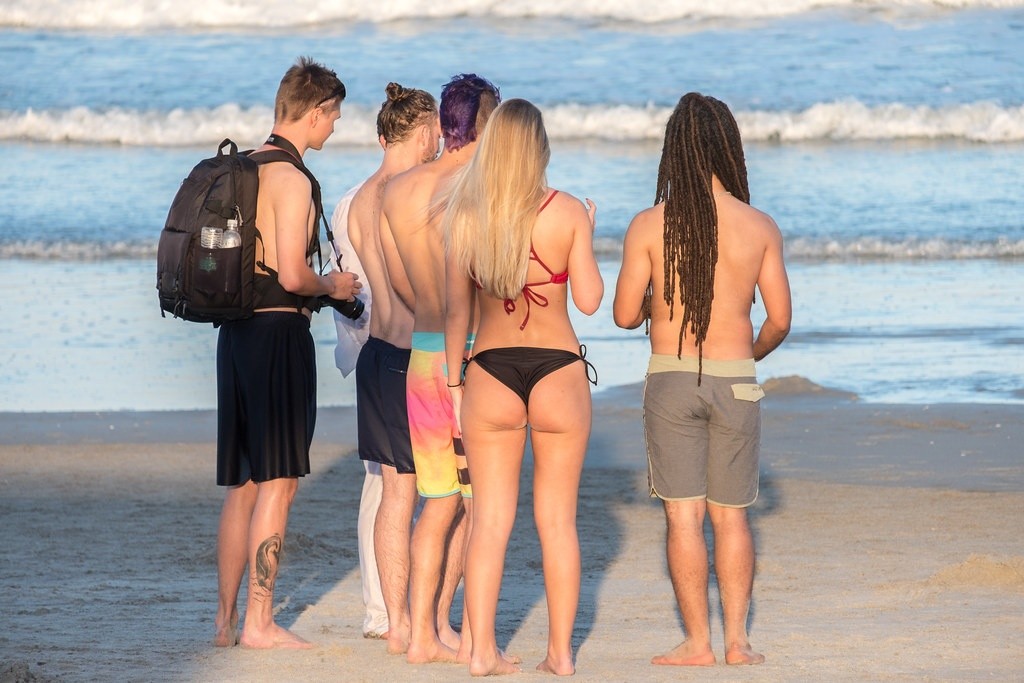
[212,56,363,651]
[612,94,790,668]
[348,75,603,677]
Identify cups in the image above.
[198,227,223,271]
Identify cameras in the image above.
[314,293,365,320]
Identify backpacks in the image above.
[155,138,322,329]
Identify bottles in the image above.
[222,219,241,248]
